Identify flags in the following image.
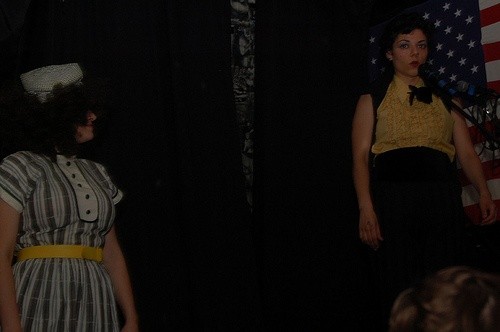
[358,0,500,225]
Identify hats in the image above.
[20,62,84,106]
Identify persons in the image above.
[390,266,500,332]
[352,14,497,332]
[0,62,139,332]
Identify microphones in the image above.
[457,80,497,99]
[416,63,457,99]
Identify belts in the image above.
[14,244,105,262]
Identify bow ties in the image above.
[406,84,433,106]
